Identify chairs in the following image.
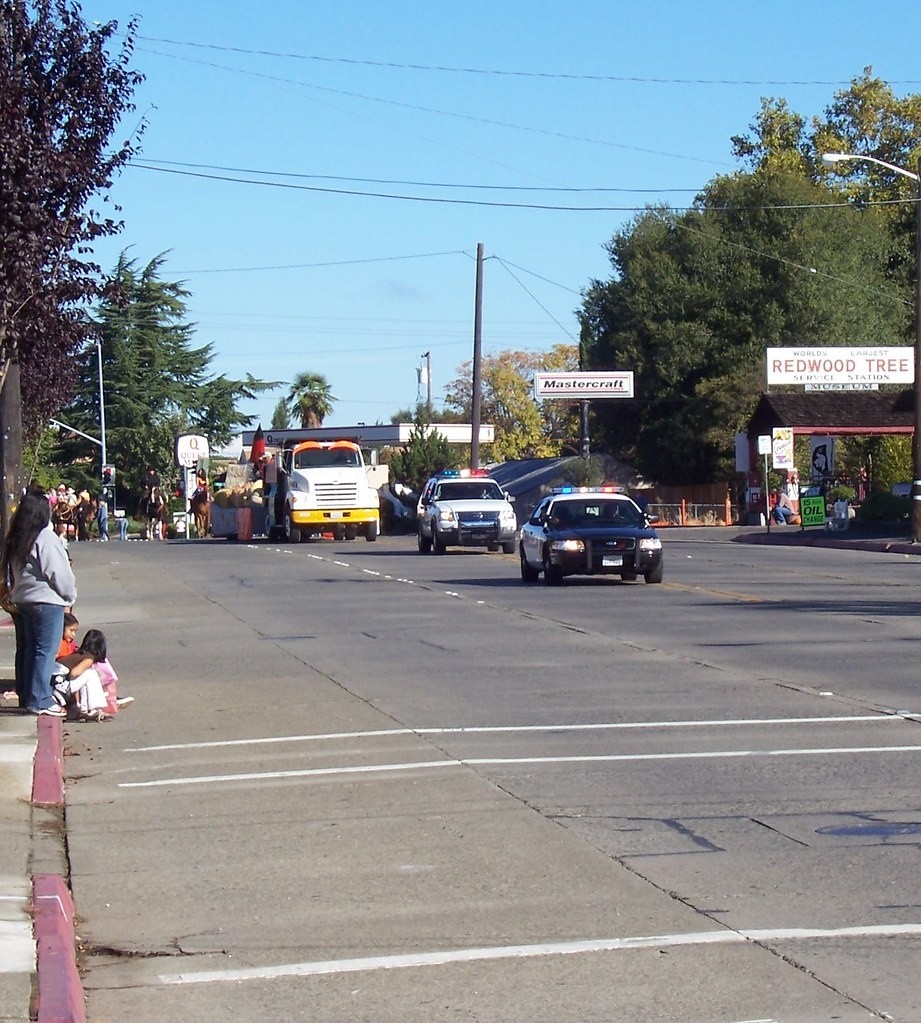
[824,499,850,532]
[556,506,569,521]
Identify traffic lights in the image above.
[103,469,111,483]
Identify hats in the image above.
[66,488,75,493]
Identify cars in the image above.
[519,486,664,587]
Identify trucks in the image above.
[273,440,389,544]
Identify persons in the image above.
[553,505,569,522]
[769,488,795,526]
[605,505,624,520]
[0,466,269,725]
[436,485,490,500]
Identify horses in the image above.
[190,486,212,539]
[145,485,169,541]
[51,492,98,542]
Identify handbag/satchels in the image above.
[50,662,72,707]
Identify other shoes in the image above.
[116,697,134,708]
[781,522,787,525]
[75,536,79,541]
[19,700,27,708]
[77,713,87,722]
[27,702,68,716]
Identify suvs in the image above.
[417,469,517,555]
